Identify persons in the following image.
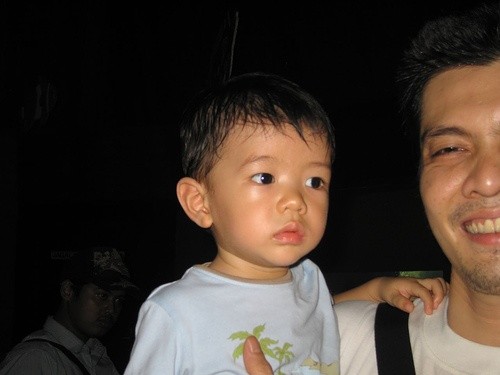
[243,15,500,375]
[123,70,450,375]
[0,246,134,374]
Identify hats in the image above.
[64,246,137,288]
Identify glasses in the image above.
[78,289,127,305]
